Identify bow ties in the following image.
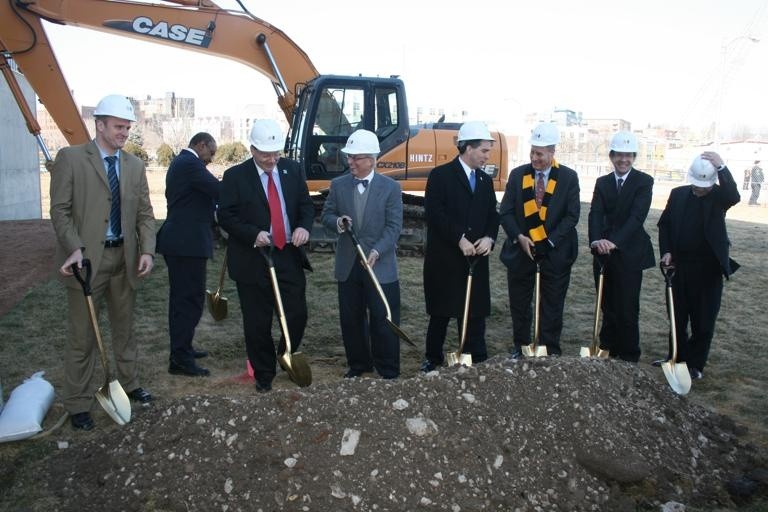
[353,177,369,187]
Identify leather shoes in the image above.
[651,358,669,367]
[419,354,445,373]
[193,350,208,358]
[167,360,211,377]
[689,367,703,379]
[256,380,272,392]
[71,411,94,431]
[343,366,374,378]
[126,387,151,402]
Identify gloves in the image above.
[530,238,553,261]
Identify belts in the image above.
[105,238,124,247]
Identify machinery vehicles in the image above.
[0,0,510,257]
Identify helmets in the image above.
[93,95,136,123]
[608,130,639,153]
[340,128,381,154]
[687,153,718,188]
[527,122,560,147]
[456,120,495,142]
[249,118,286,153]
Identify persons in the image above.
[748,159,764,206]
[155,132,220,378]
[49,92,157,426]
[652,151,742,382]
[587,130,657,364]
[217,119,316,392]
[500,122,581,358]
[418,120,500,371]
[743,167,751,190]
[320,130,403,380]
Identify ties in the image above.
[535,173,545,209]
[264,171,286,251]
[616,179,623,195]
[468,170,476,192]
[104,156,122,237]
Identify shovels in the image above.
[341,218,419,347]
[520,251,548,357]
[206,244,229,322]
[578,250,610,358]
[255,243,313,389]
[446,252,479,366]
[660,260,692,396]
[71,257,132,427]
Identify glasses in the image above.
[347,154,370,160]
